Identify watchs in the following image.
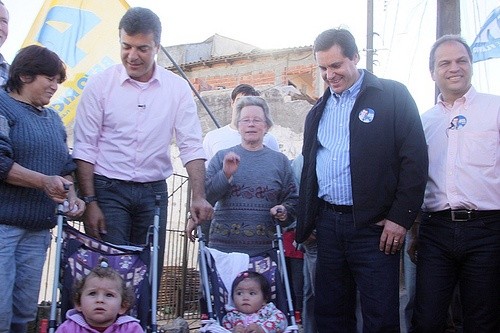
[82,195,98,203]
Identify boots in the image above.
[11,318,37,333]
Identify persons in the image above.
[188,83,279,243]
[72,6,214,329]
[0,0,12,85]
[297,28,429,333]
[221,270,288,333]
[195,97,297,310]
[407,34,500,333]
[0,45,85,333]
[54,266,146,333]
[276,152,318,333]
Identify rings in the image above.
[394,240,399,243]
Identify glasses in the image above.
[237,118,266,125]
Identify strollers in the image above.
[196,209,299,333]
[36,184,163,333]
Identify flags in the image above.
[16,0,133,126]
[468,5,500,62]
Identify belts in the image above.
[321,204,352,213]
[432,210,500,221]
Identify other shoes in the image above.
[294,311,301,322]
